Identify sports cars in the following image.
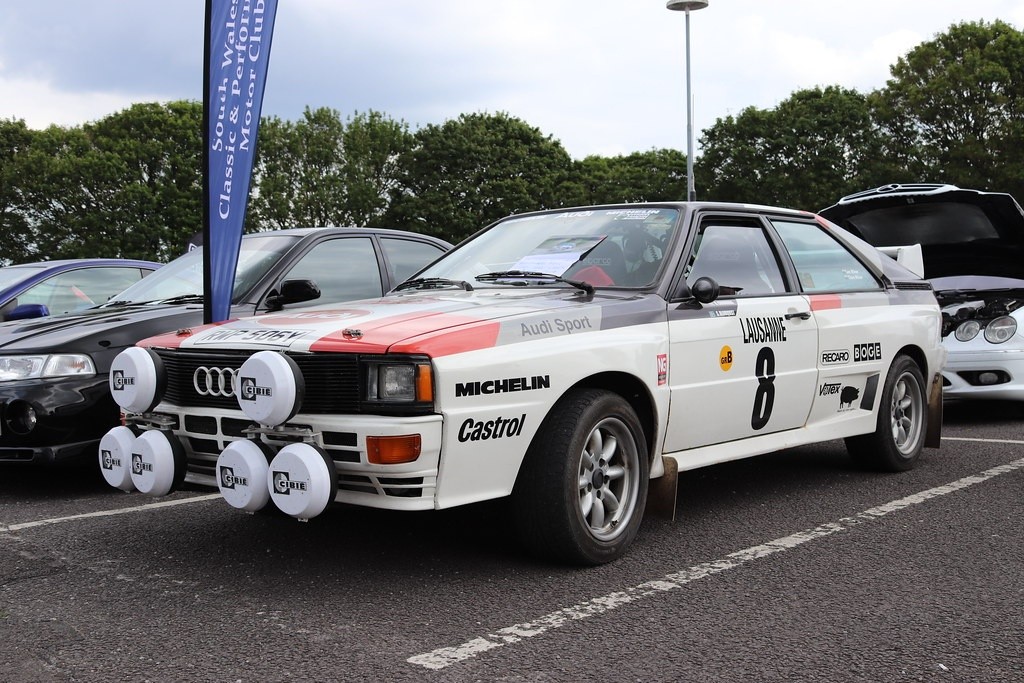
[98,197,946,565]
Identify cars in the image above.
[0,224,508,486]
[0,258,207,322]
[814,180,1024,405]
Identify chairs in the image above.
[562,241,627,281]
[699,236,763,285]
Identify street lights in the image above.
[664,0,708,202]
